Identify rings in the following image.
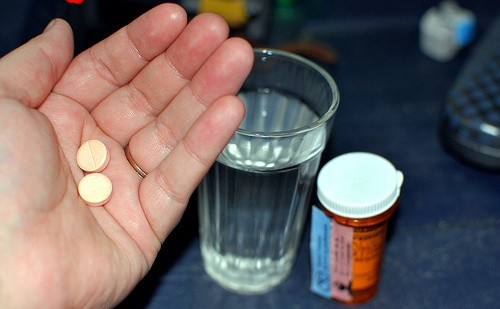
[126,146,148,178]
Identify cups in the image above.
[197,47,340,296]
